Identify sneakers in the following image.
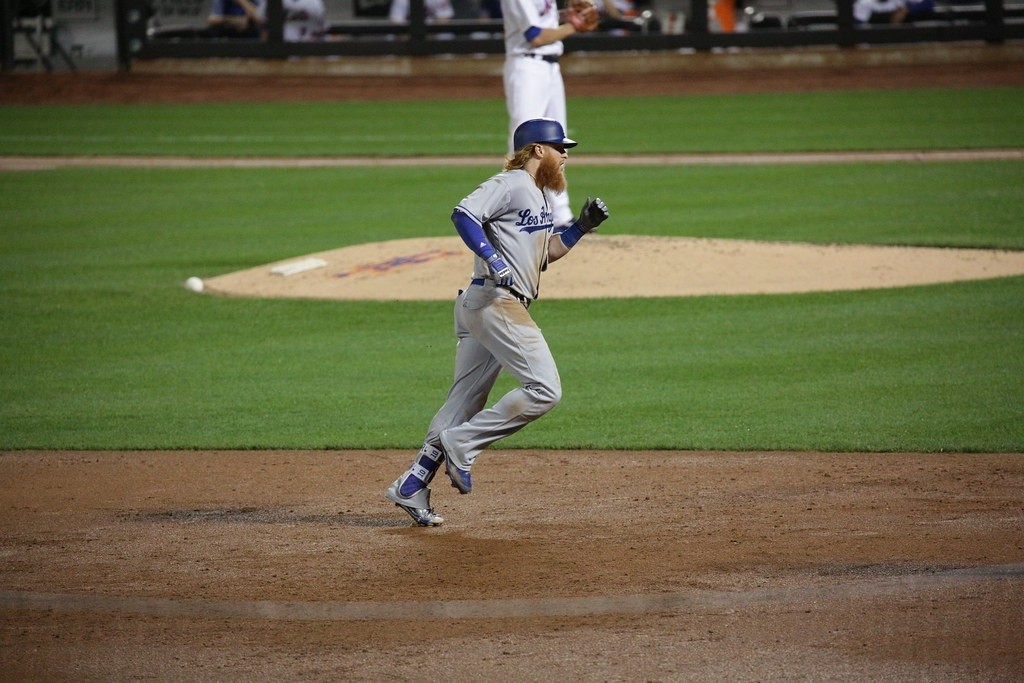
[385,471,444,528]
[438,433,472,494]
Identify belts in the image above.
[471,278,531,305]
[524,53,560,64]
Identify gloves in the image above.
[485,249,515,287]
[574,196,609,234]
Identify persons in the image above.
[237,0,635,55]
[503,0,602,234]
[386,117,611,527]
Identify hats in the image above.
[514,119,578,151]
